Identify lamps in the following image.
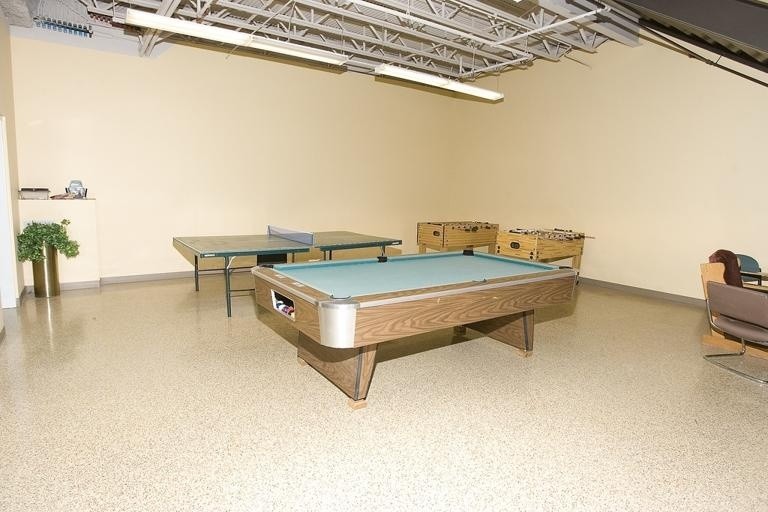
[125,8,348,66]
[375,63,505,102]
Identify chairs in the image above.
[703,280,768,384]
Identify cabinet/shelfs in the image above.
[495,229,585,269]
[417,220,499,255]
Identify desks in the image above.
[252,249,581,409]
[699,258,768,359]
[173,230,402,317]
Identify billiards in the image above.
[276,300,294,318]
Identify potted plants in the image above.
[16,218,79,298]
